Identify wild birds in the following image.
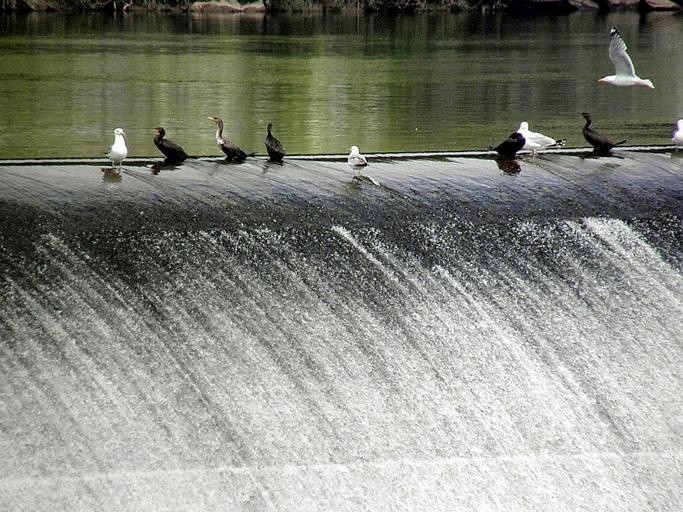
[348,145,367,177]
[669,119,683,150]
[205,116,247,161]
[598,24,655,89]
[517,121,557,156]
[264,124,286,161]
[488,132,526,158]
[576,109,627,154]
[100,128,128,169]
[148,127,189,162]
